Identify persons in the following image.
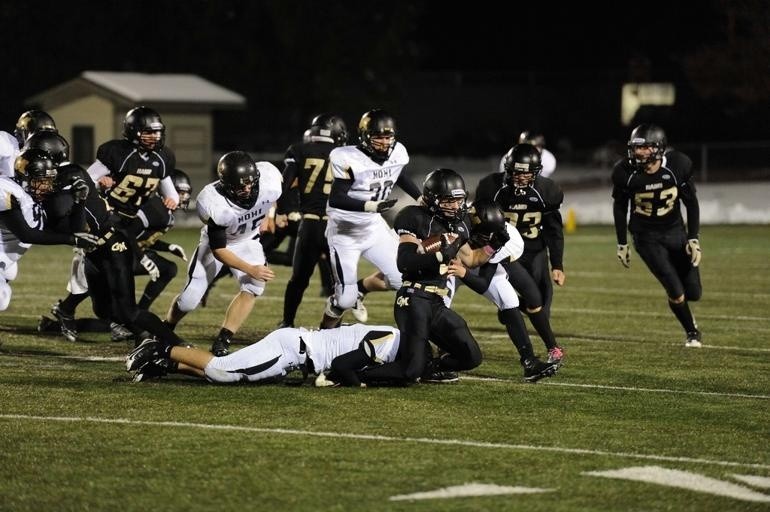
[2,106,564,386]
[612,124,702,349]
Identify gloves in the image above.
[683,237,706,269]
[363,194,400,216]
[73,230,101,253]
[168,242,189,264]
[138,253,162,283]
[486,227,511,251]
[437,232,470,270]
[614,242,634,272]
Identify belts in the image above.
[81,224,117,255]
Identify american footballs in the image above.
[416,232,459,255]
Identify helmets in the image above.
[467,197,510,247]
[502,141,543,179]
[516,128,548,148]
[628,121,670,153]
[120,103,168,141]
[309,110,349,144]
[158,166,194,197]
[27,130,72,165]
[15,149,60,184]
[355,107,399,147]
[14,109,60,135]
[214,150,262,189]
[419,166,469,211]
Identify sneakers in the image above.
[683,328,705,349]
[108,321,136,343]
[131,353,171,385]
[518,354,560,382]
[49,301,82,343]
[125,337,161,372]
[546,346,565,366]
[421,369,461,385]
[211,334,233,358]
[350,288,372,323]
